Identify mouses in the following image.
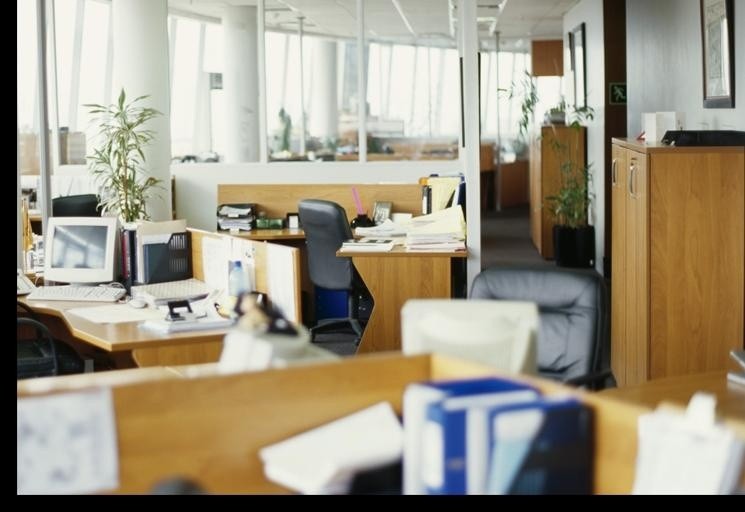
[130,298,147,308]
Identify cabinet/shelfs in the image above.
[611,136,744,390]
[496,161,529,215]
[526,125,587,262]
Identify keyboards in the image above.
[27,286,125,302]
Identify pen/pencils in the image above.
[353,187,363,215]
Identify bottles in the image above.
[229,260,245,296]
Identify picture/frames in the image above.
[569,21,587,112]
[698,0,736,110]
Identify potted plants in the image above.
[496,66,600,269]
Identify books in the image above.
[216,202,267,231]
[123,218,237,334]
[399,373,579,497]
[342,175,467,255]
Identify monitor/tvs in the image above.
[44,216,120,284]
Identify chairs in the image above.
[13,312,84,380]
[54,193,102,216]
[298,198,377,348]
[466,262,618,397]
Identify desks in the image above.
[228,223,322,341]
[18,278,268,367]
[337,212,468,355]
